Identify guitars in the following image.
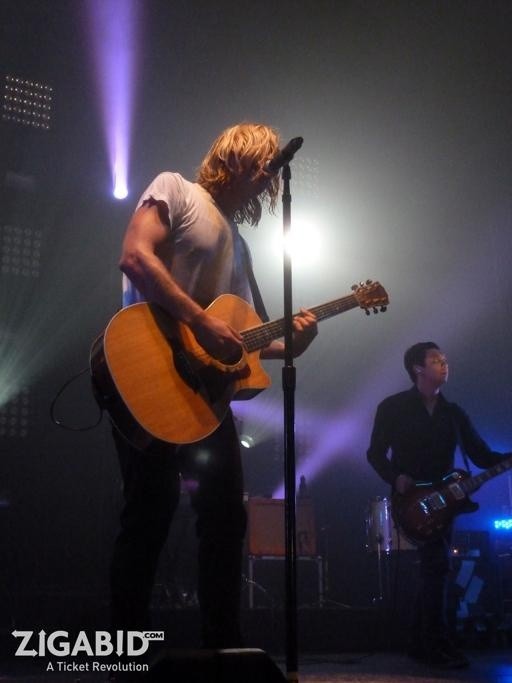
[390,451,512,544]
[87,278,390,455]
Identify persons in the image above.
[101,119,322,646]
[366,338,510,671]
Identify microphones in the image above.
[262,136,304,176]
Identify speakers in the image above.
[140,647,287,683]
